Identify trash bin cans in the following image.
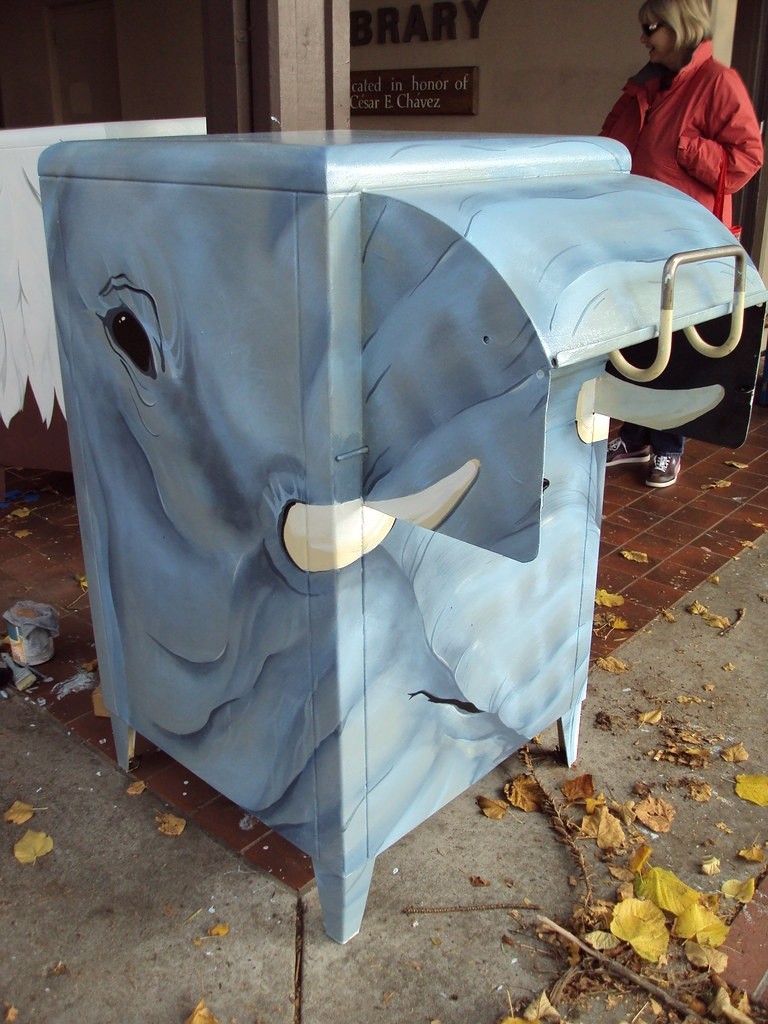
[35,125,767,952]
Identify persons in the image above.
[597,0,763,488]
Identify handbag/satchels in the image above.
[713,147,742,242]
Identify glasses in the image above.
[642,21,666,37]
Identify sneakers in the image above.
[645,454,681,488]
[606,437,651,466]
